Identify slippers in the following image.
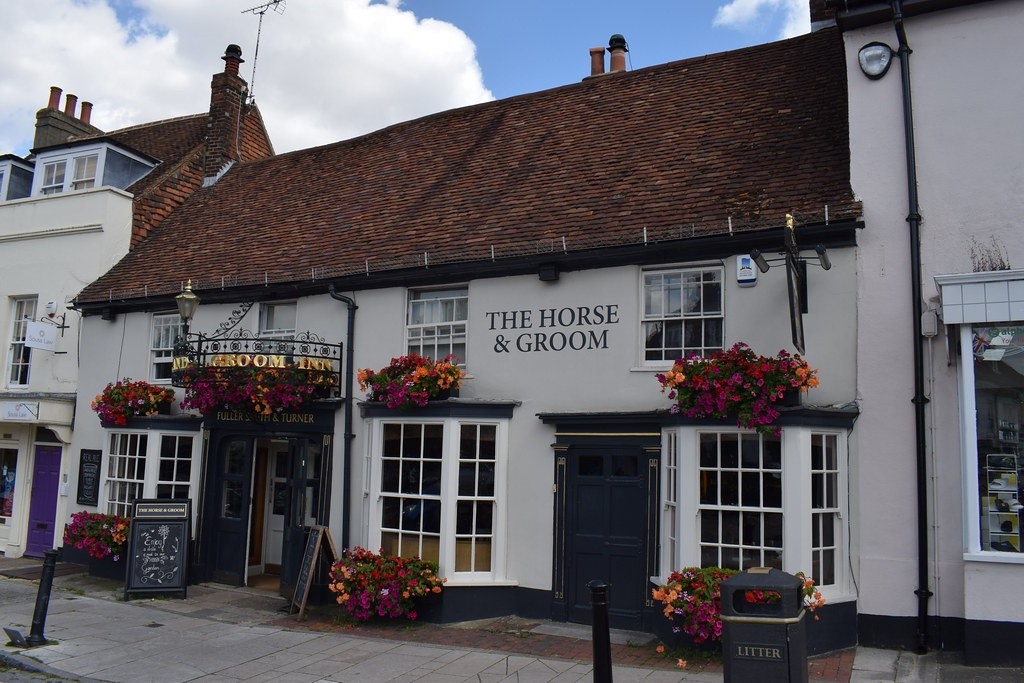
[990,478,1008,489]
[991,541,1020,552]
[989,458,1015,468]
[1001,521,1012,532]
[995,499,1009,512]
[1008,499,1024,510]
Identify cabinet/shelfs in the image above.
[986,454,1020,551]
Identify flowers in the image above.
[356,351,465,407]
[179,354,331,414]
[327,546,446,621]
[62,510,130,561]
[653,340,818,437]
[90,381,174,425]
[651,567,825,666]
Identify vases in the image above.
[675,611,694,636]
[677,387,802,406]
[106,538,128,554]
[156,401,170,414]
[304,387,329,399]
[428,381,458,399]
[412,589,443,601]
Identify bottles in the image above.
[999,419,1016,440]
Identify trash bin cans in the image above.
[716,567,809,683]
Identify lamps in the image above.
[857,41,892,79]
[749,248,785,272]
[174,278,200,335]
[794,244,831,270]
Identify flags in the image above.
[972,328,994,356]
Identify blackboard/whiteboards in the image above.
[292,524,323,608]
[75,448,103,507]
[131,521,185,589]
